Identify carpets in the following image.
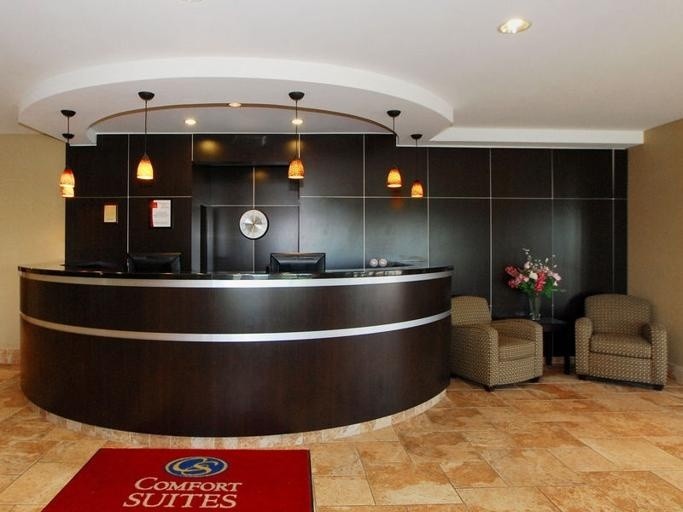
[42,447,315,512]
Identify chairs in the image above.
[449,293,545,392]
[574,293,668,392]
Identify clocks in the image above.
[238,208,269,241]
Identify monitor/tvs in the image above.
[271,252,326,275]
[124,252,181,274]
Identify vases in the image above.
[526,295,543,323]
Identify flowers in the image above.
[505,247,562,312]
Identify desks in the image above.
[508,316,572,383]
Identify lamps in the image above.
[136,91,155,180]
[287,92,305,180]
[386,109,403,187]
[57,108,79,188]
[60,132,77,196]
[410,132,425,198]
